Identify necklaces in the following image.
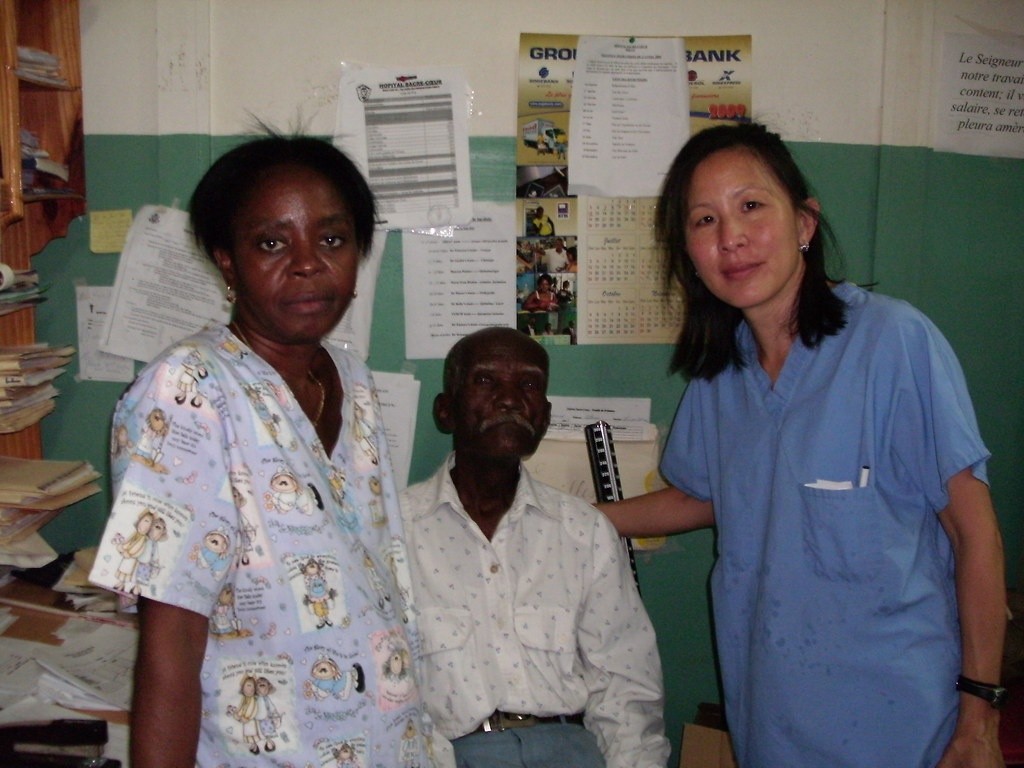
[230,319,326,436]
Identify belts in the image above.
[472,708,586,732]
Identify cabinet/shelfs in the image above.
[1,0,91,464]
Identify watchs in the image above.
[954,673,1008,711]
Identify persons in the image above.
[516,207,577,312]
[587,118,1008,768]
[389,325,676,767]
[90,105,429,768]
